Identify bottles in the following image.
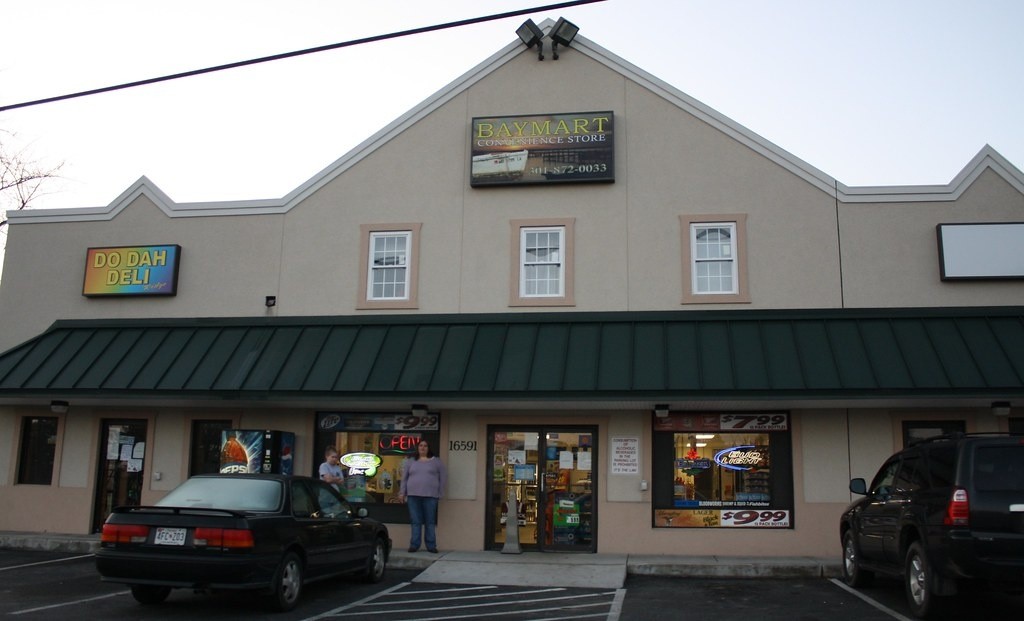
[263,449,272,473]
[219,430,250,477]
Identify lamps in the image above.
[991,401,1009,416]
[412,404,428,416]
[655,404,669,417]
[50,400,69,412]
[516,18,545,61]
[548,17,579,60]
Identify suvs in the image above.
[840,432,1024,621]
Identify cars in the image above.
[96,472,390,614]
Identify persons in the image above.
[318,444,344,493]
[397,438,446,553]
